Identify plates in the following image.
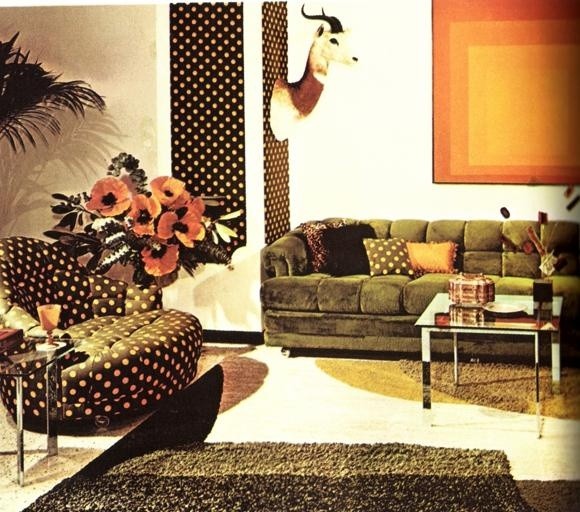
[482,302,527,314]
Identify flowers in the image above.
[42,149,244,284]
[500,188,580,279]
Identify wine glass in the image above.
[36,303,61,348]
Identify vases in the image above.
[533,280,553,327]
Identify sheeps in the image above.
[268,3,358,144]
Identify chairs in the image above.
[0,236,203,433]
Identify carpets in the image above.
[17,350,580,512]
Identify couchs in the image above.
[259,217,580,357]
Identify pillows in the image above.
[303,222,460,277]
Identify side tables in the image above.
[0,338,84,486]
